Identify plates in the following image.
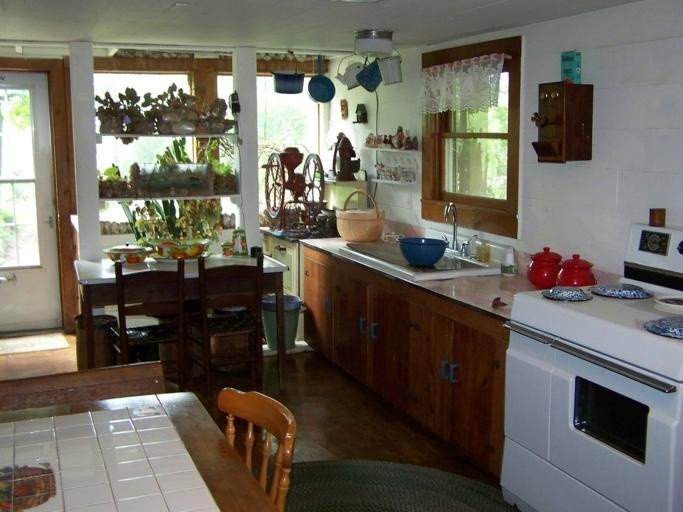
[541,281,651,301]
[644,315,682,339]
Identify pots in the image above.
[103,241,151,265]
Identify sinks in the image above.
[337,239,502,282]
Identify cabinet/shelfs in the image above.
[97,131,238,203]
[301,246,333,359]
[363,146,418,186]
[261,236,298,298]
[532,80,593,164]
[331,260,507,474]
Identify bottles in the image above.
[363,125,418,151]
[376,156,417,183]
[353,104,366,123]
[501,247,516,277]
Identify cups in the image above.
[649,208,666,226]
[220,240,233,256]
[474,245,491,262]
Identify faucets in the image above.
[443,201,462,250]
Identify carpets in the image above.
[0,332,70,356]
[259,457,518,511]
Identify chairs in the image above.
[99,254,264,400]
[214,384,297,512]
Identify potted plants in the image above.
[94,81,235,135]
[100,139,236,197]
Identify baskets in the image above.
[336,190,384,242]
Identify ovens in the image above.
[498,313,682,496]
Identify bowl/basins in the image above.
[397,236,447,268]
[147,238,210,258]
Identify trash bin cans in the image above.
[262,295,301,351]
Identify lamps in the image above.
[333,27,403,91]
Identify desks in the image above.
[74,259,288,369]
[0,392,296,510]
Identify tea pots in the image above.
[335,54,370,89]
